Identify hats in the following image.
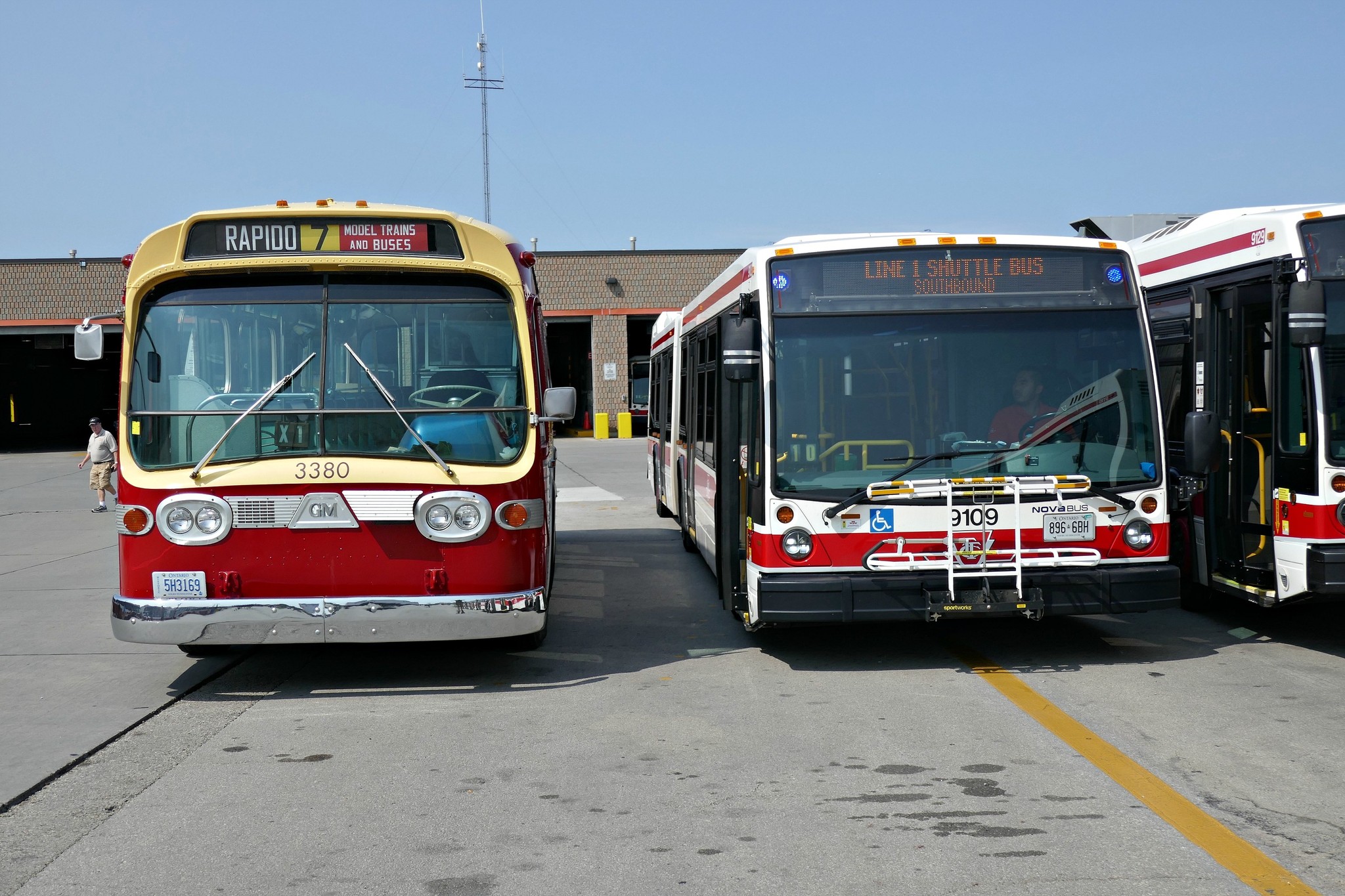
[89,417,100,426]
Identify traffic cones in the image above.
[583,412,592,430]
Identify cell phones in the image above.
[111,467,114,473]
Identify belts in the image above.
[94,459,114,464]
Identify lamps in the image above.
[607,278,617,283]
[79,260,86,266]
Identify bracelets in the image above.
[115,462,118,464]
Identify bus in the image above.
[1124,204,1344,610]
[73,199,578,659]
[647,232,1216,646]
[629,355,650,434]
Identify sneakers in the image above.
[115,497,118,505]
[92,505,108,512]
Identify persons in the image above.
[78,417,118,513]
[990,365,1077,445]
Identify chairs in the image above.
[282,368,496,450]
